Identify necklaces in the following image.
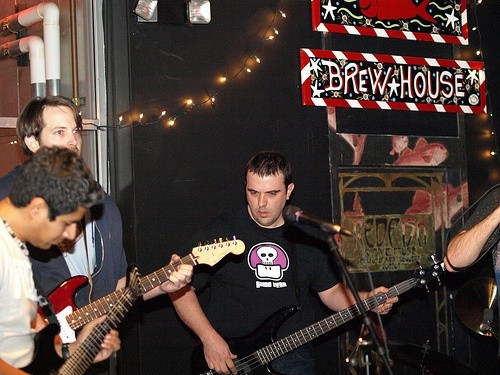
[0,214,29,256]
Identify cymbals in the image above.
[453,275,500,336]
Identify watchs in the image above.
[62,343,70,359]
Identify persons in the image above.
[445,187,500,302]
[0,145,121,375]
[0,96,128,310]
[167,152,398,375]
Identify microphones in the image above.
[282,206,355,238]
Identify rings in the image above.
[186,278,191,283]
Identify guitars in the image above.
[190,252,447,375]
[18,262,145,375]
[36,236,245,346]
